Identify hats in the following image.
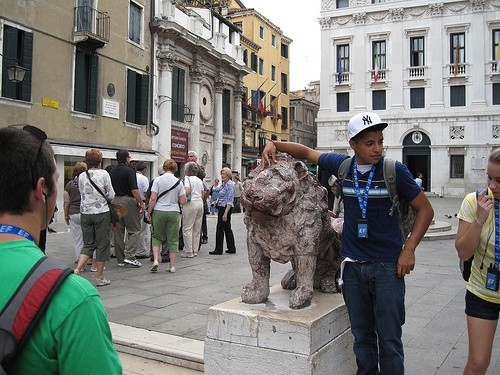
[137,161,147,171]
[348,112,388,140]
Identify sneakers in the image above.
[118,259,142,267]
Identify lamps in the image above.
[0,54,27,83]
[158,95,195,123]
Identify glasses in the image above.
[8,125,47,189]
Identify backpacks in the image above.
[459,190,488,282]
[338,158,416,245]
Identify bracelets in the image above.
[475,217,485,227]
[270,139,277,148]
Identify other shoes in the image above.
[151,257,170,263]
[95,279,111,286]
[164,267,176,273]
[79,265,86,271]
[226,250,236,253]
[181,253,198,258]
[90,267,106,272]
[150,263,158,271]
[209,250,221,255]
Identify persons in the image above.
[455,148,500,375]
[0,124,124,375]
[109,150,145,267]
[74,148,115,286]
[210,175,246,214]
[261,113,434,375]
[188,151,209,244]
[209,168,237,256]
[414,172,425,191]
[181,162,206,257]
[105,159,169,261]
[64,162,93,265]
[148,159,186,273]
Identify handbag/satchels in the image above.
[145,206,152,224]
[109,204,121,224]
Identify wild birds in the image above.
[454,212,458,218]
[444,213,453,219]
